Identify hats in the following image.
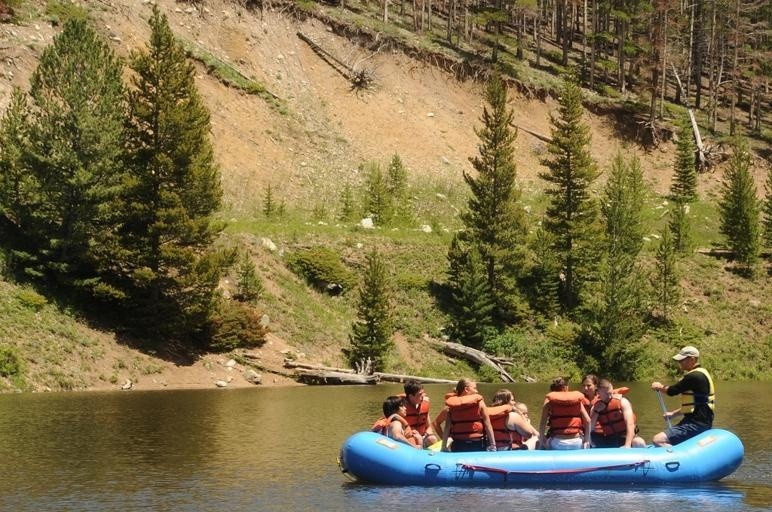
[672,345,699,361]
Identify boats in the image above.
[335,429,747,491]
[341,480,747,510]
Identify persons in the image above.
[367,369,647,453]
[648,344,717,448]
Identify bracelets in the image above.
[661,385,668,392]
